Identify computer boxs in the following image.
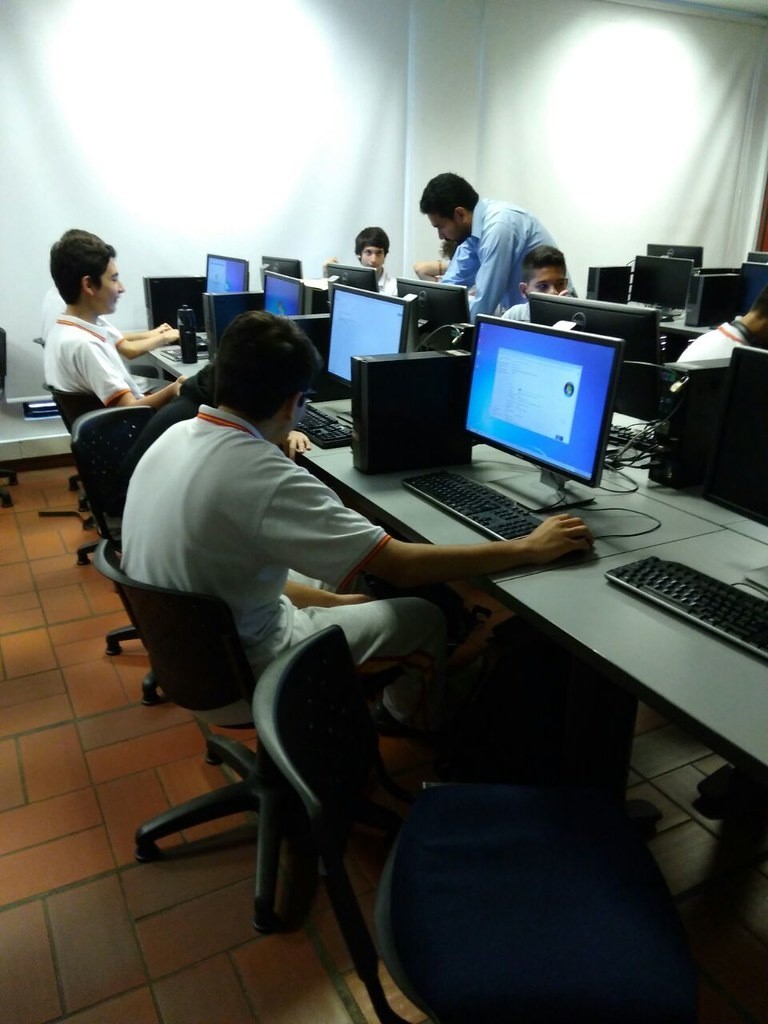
[685,274,740,327]
[144,277,205,333]
[586,266,631,305]
[279,312,351,403]
[351,350,471,476]
[203,291,265,362]
[648,358,731,490]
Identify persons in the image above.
[413,174,577,325]
[502,245,569,323]
[42,230,190,407]
[117,360,311,487]
[117,310,595,731]
[676,285,768,362]
[323,227,398,296]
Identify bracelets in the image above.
[439,260,441,275]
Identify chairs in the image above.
[39,382,105,522]
[70,404,288,933]
[0,329,17,508]
[253,624,708,1024]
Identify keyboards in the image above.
[401,470,545,543]
[607,425,655,453]
[291,403,352,449]
[604,556,768,661]
[171,333,203,347]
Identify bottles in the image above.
[177,305,196,363]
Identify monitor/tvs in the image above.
[202,244,768,591]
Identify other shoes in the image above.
[372,699,425,740]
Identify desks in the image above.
[150,317,768,769]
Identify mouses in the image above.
[197,343,208,351]
[660,314,672,319]
